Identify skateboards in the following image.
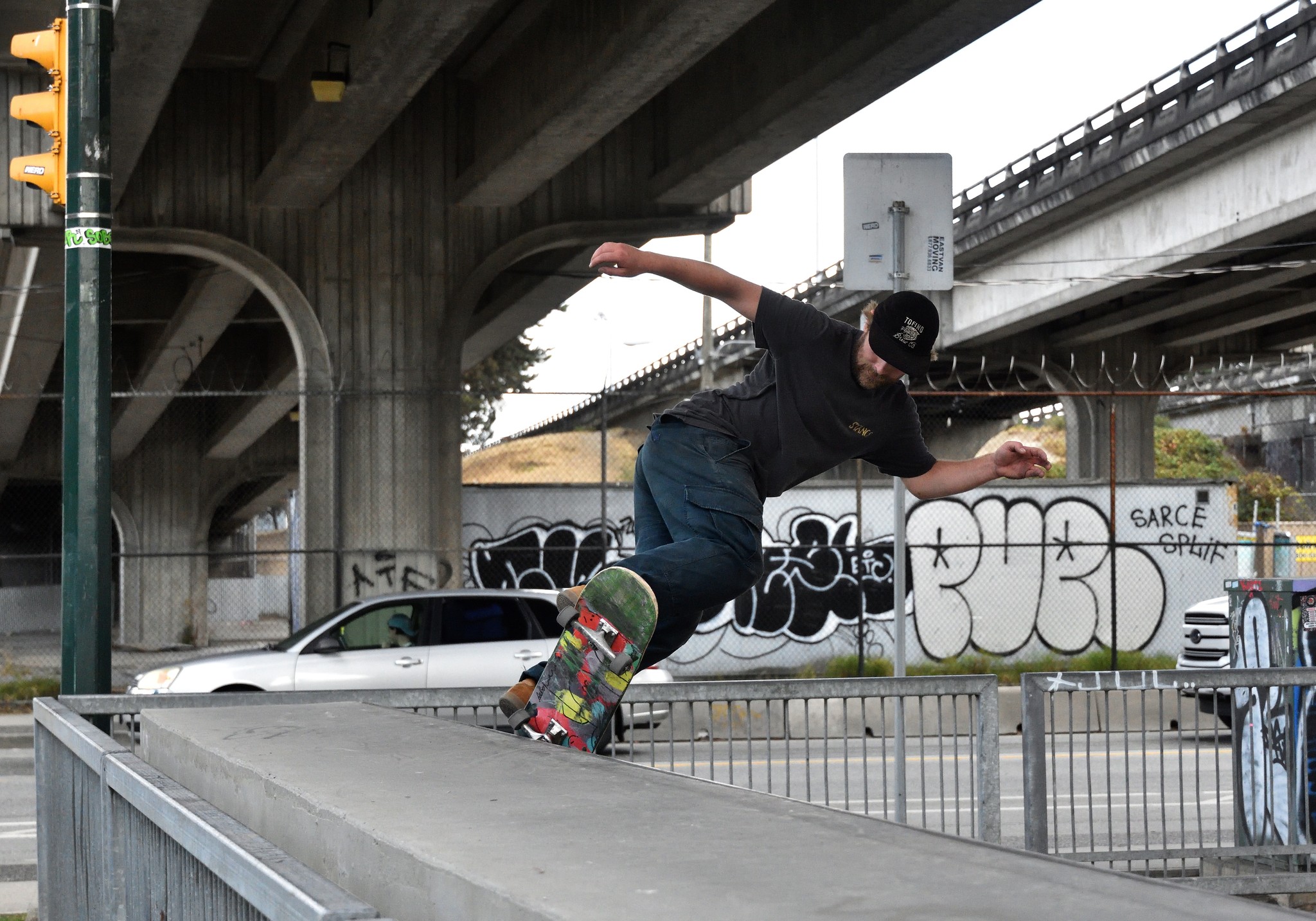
[507,565,658,753]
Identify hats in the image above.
[387,613,416,636]
[868,291,939,379]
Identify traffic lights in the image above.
[10,17,69,207]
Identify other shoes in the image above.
[499,681,536,717]
[555,583,588,612]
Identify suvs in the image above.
[118,588,675,755]
[1174,596,1233,730]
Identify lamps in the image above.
[289,405,300,421]
[308,41,352,103]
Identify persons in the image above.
[380,613,417,649]
[499,242,1052,718]
